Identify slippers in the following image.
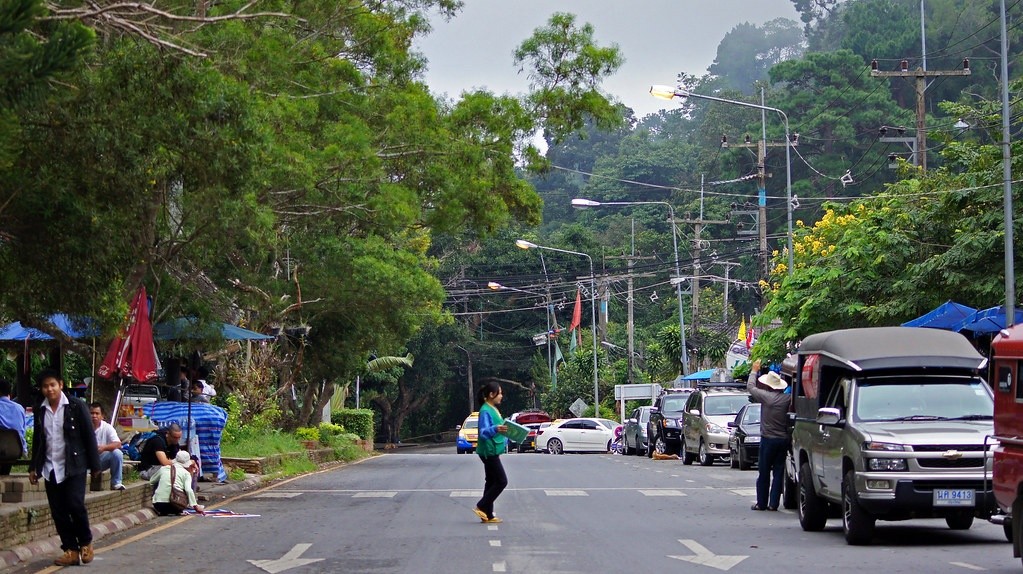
[480,518,503,523]
[472,508,488,521]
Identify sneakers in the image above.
[80,542,94,564]
[54,549,79,566]
[112,483,126,490]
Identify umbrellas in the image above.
[0,296,274,409]
[96,282,161,426]
[681,367,719,379]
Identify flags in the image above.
[738,316,747,342]
[551,343,562,390]
[569,289,582,357]
[746,318,755,348]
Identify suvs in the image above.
[680,379,753,466]
[647,388,696,459]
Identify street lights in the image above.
[487,282,552,378]
[445,341,474,414]
[648,84,795,276]
[570,198,690,387]
[515,240,599,418]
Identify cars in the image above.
[535,418,620,455]
[454,411,551,454]
[621,405,653,456]
[117,384,162,414]
[727,403,762,470]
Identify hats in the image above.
[172,451,192,468]
[758,371,788,390]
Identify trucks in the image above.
[784,327,994,545]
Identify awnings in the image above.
[901,300,1022,335]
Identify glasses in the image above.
[169,430,181,441]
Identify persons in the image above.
[746,358,791,511]
[28,367,103,566]
[88,402,127,491]
[0,380,34,478]
[138,423,182,480]
[167,359,216,404]
[472,381,508,523]
[150,450,206,516]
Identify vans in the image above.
[981,319,1023,558]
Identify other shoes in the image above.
[752,503,766,511]
[767,505,777,511]
[177,512,187,516]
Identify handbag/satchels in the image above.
[170,488,188,509]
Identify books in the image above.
[498,418,531,444]
